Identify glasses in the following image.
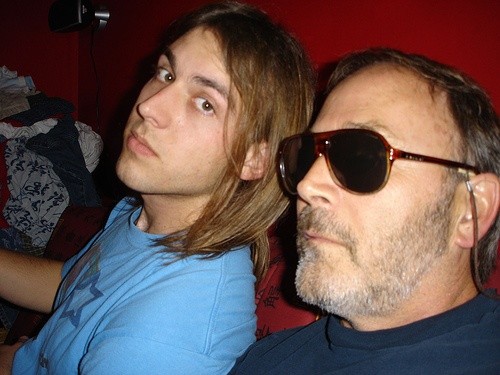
[275,128,478,195]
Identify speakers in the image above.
[49,0,95,35]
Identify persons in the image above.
[1,5,313,375]
[224,47,500,374]
